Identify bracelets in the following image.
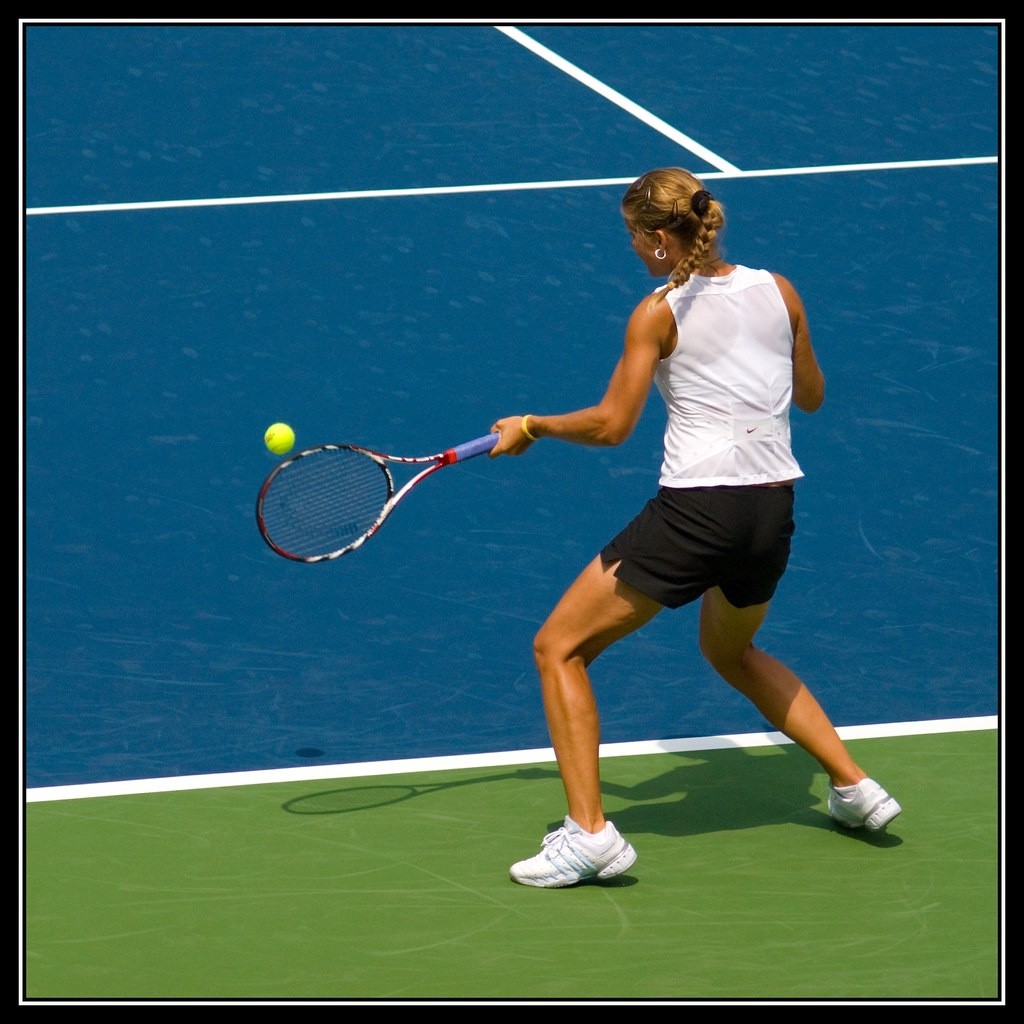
[522,414,540,441]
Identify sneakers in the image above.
[508,815,638,890]
[827,776,903,833]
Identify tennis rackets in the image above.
[257,431,500,563]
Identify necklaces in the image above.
[705,257,722,266]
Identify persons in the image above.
[488,166,901,888]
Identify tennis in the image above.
[264,423,295,455]
